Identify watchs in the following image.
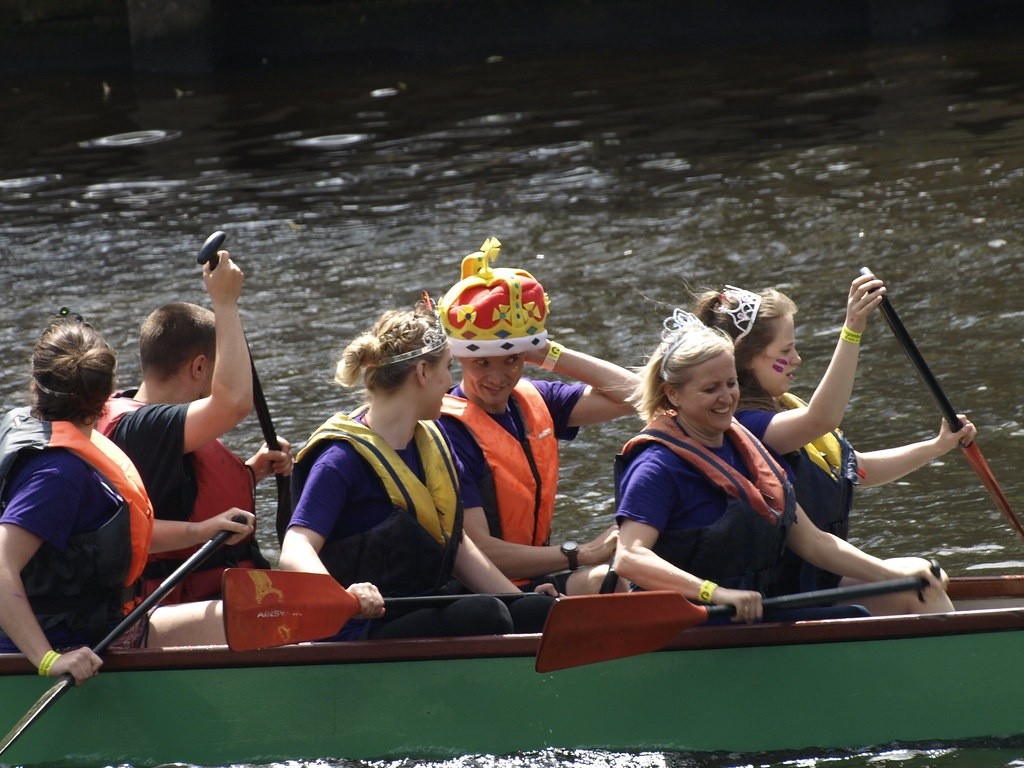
[559,539,579,571]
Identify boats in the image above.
[0,575,1024,767]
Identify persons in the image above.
[275,249,646,635]
[602,273,979,616]
[1,251,294,685]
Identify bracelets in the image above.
[538,341,564,373]
[696,580,718,604]
[37,650,62,677]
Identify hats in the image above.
[438,237,550,357]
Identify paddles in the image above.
[534,555,945,676]
[598,550,619,594]
[196,231,310,552]
[0,514,250,757]
[859,264,1024,548]
[219,565,560,653]
